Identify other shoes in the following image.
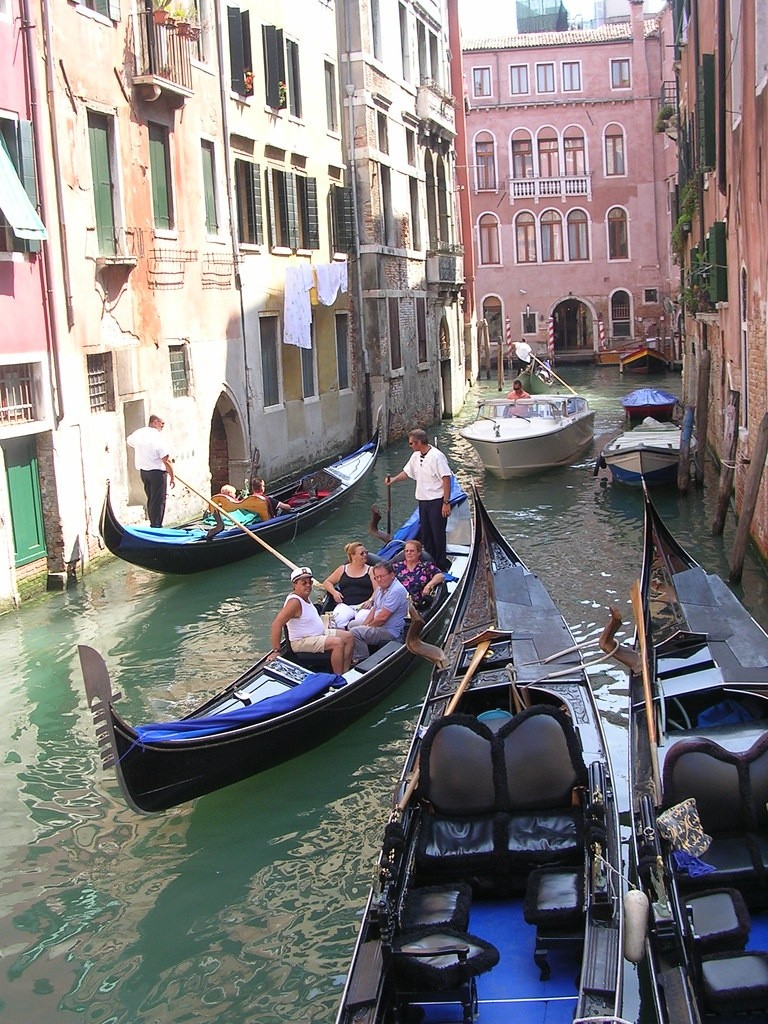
[350,659,365,666]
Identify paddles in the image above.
[172,473,321,586]
[530,353,577,395]
[386,473,391,533]
[397,624,497,813]
[630,578,663,808]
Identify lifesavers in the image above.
[593,451,607,476]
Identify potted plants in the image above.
[152,0,209,42]
[656,108,678,133]
[678,212,690,232]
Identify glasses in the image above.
[404,549,417,553]
[514,387,520,390]
[297,580,313,585]
[410,441,415,445]
[352,550,368,556]
[156,422,165,425]
[374,573,389,581]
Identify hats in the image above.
[291,567,313,582]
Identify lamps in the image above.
[541,315,544,321]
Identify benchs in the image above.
[380,700,592,1023]
[283,546,435,659]
[663,734,767,1023]
[207,493,273,522]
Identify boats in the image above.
[620,389,677,421]
[514,352,555,395]
[75,435,474,816]
[98,404,382,573]
[598,415,699,491]
[597,350,636,365]
[618,345,669,375]
[618,476,767,1024]
[458,392,594,481]
[335,478,624,1023]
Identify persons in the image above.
[221,484,239,501]
[126,415,175,528]
[385,428,452,573]
[253,479,291,516]
[391,539,443,613]
[348,561,409,663]
[514,338,531,376]
[506,380,533,419]
[324,542,379,631]
[267,567,355,677]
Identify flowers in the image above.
[277,82,286,111]
[239,66,255,98]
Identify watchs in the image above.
[273,649,280,652]
[442,500,450,505]
